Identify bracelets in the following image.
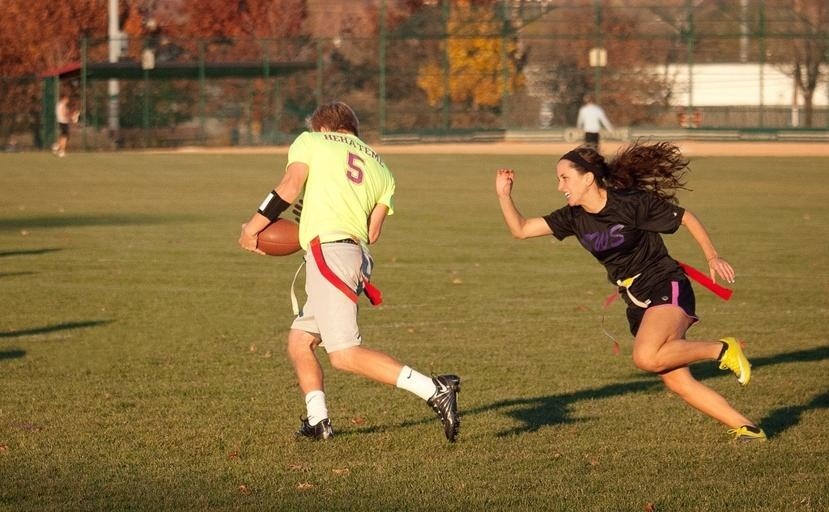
[707,255,718,261]
[257,190,291,223]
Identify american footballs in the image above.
[256,219,302,255]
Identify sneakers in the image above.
[716,335,752,388]
[727,427,767,443]
[294,416,332,441]
[427,374,460,441]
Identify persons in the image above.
[51,95,77,158]
[496,136,768,445]
[238,102,461,444]
[576,94,612,148]
